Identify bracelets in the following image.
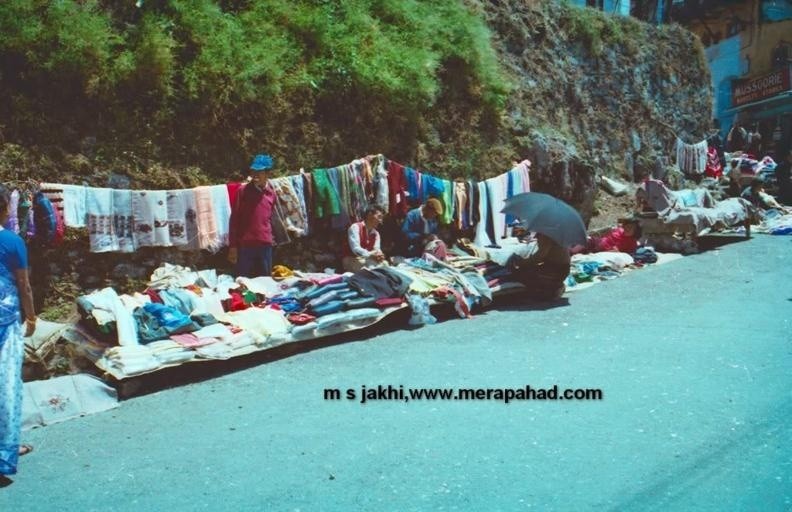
[25,316,38,324]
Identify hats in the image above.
[427,198,443,216]
[250,154,273,171]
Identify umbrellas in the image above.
[499,189,589,250]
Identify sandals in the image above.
[18,445,33,456]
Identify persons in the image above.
[342,205,389,274]
[726,160,742,198]
[0,190,39,486]
[571,216,642,253]
[226,154,277,277]
[636,171,671,211]
[741,132,762,161]
[774,149,792,206]
[400,197,444,257]
[739,177,789,226]
[513,232,570,301]
